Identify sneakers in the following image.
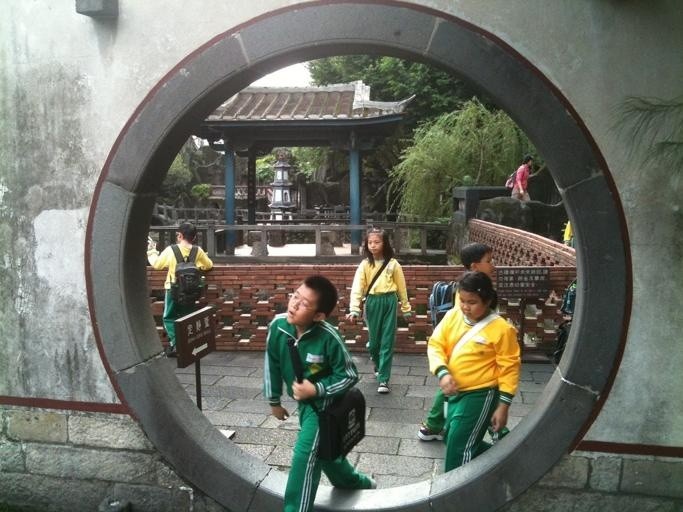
[378,381,388,393]
[375,368,378,377]
[166,345,176,357]
[418,423,446,441]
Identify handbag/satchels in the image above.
[318,388,365,460]
[553,320,571,363]
[560,278,576,314]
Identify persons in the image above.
[416,242,511,444]
[144,223,215,356]
[511,153,535,203]
[426,271,522,474]
[260,272,376,512]
[348,227,415,394]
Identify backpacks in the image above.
[505,165,525,189]
[429,280,458,329]
[170,244,208,305]
